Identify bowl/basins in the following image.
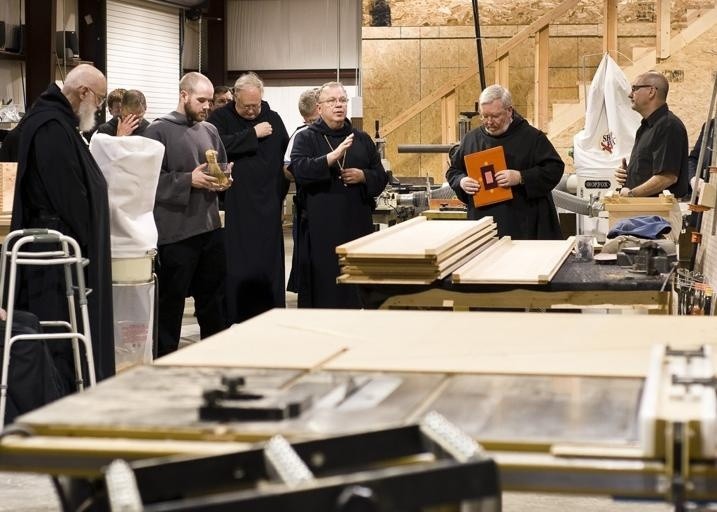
[204,163,231,187]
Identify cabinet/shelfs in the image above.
[225,1,363,80]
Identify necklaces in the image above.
[323,134,348,187]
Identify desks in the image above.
[328,228,680,314]
[4,308,713,508]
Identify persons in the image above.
[97,88,127,134]
[101,89,150,137]
[291,82,388,309]
[143,71,234,359]
[2,64,116,388]
[615,72,689,198]
[286,88,320,293]
[209,85,233,111]
[445,84,565,239]
[205,74,291,328]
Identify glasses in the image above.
[89,87,106,107]
[319,95,350,105]
[632,84,657,92]
